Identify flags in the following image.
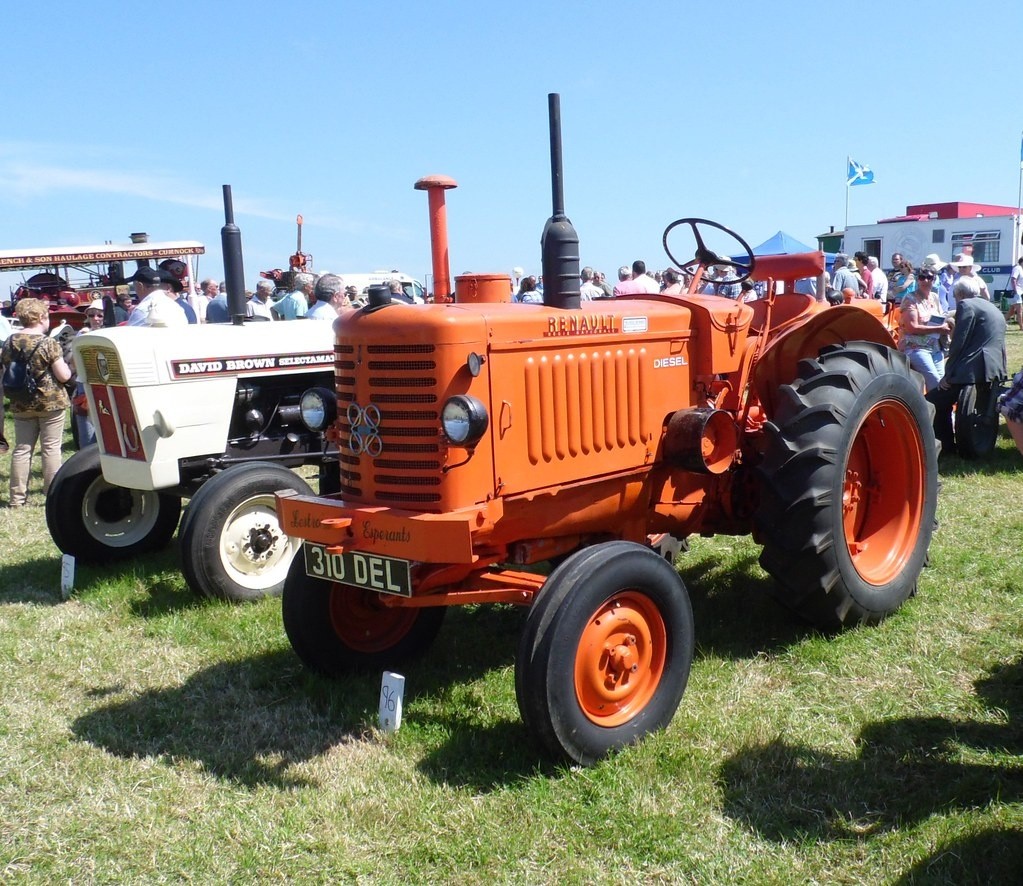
[847,160,877,186]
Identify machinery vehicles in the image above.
[283,93,941,769]
[46,185,339,604]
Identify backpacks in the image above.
[3,334,51,405]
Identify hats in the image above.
[84,299,103,316]
[122,265,160,284]
[950,254,981,273]
[715,256,732,272]
[156,269,182,292]
[920,254,947,273]
[847,259,859,272]
[949,261,959,273]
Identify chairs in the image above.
[742,250,825,339]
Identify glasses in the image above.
[900,265,906,269]
[957,265,966,268]
[87,313,104,319]
[834,260,842,262]
[594,277,599,279]
[918,274,933,281]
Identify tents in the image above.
[730,230,836,274]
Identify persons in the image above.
[613,260,660,296]
[3,298,72,508]
[795,277,816,296]
[0,314,12,452]
[70,266,232,448]
[646,256,758,302]
[269,273,313,321]
[303,273,345,319]
[336,286,359,315]
[388,280,413,304]
[937,275,1008,458]
[246,281,280,321]
[580,267,613,300]
[510,275,543,302]
[996,369,1023,453]
[1004,256,1023,331]
[825,251,990,339]
[897,267,955,427]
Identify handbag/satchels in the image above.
[1002,288,1015,297]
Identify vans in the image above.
[318,267,429,305]
